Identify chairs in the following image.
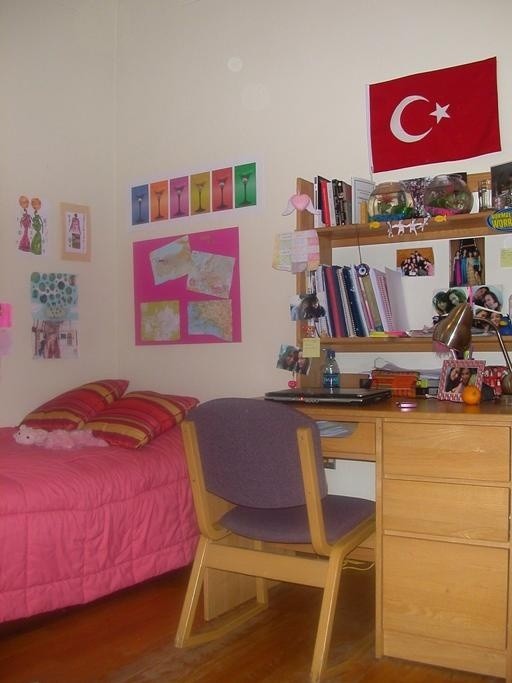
[173,398,376,683]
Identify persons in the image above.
[476,309,490,327]
[445,287,466,311]
[465,250,477,285]
[293,348,311,374]
[480,290,502,313]
[398,249,433,277]
[454,367,477,394]
[451,249,462,287]
[459,248,469,286]
[37,328,62,360]
[277,344,299,371]
[431,291,455,317]
[471,250,483,285]
[443,367,463,393]
[490,311,504,328]
[468,285,490,308]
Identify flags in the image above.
[365,54,502,174]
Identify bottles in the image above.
[322,350,340,388]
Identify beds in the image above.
[0,422,201,625]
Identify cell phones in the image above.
[400,398,418,409]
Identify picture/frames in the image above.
[437,360,486,403]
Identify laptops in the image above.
[264,387,393,407]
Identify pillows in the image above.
[79,390,200,449]
[17,380,129,431]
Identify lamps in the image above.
[431,303,512,375]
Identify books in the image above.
[305,261,409,337]
[309,175,375,228]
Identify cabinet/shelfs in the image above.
[296,173,512,683]
